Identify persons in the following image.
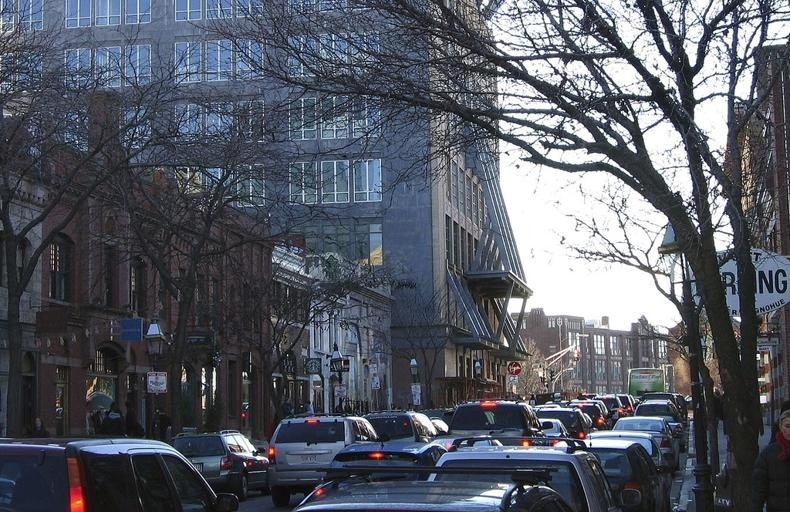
[713,389,726,436]
[392,398,469,411]
[335,399,369,417]
[23,417,50,438]
[89,400,173,442]
[756,412,765,457]
[281,396,315,418]
[769,400,790,442]
[500,392,588,405]
[748,410,790,511]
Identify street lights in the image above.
[331,344,345,413]
[659,189,720,512]
[144,315,167,443]
[409,356,420,409]
[476,362,483,399]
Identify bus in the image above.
[628,368,664,396]
